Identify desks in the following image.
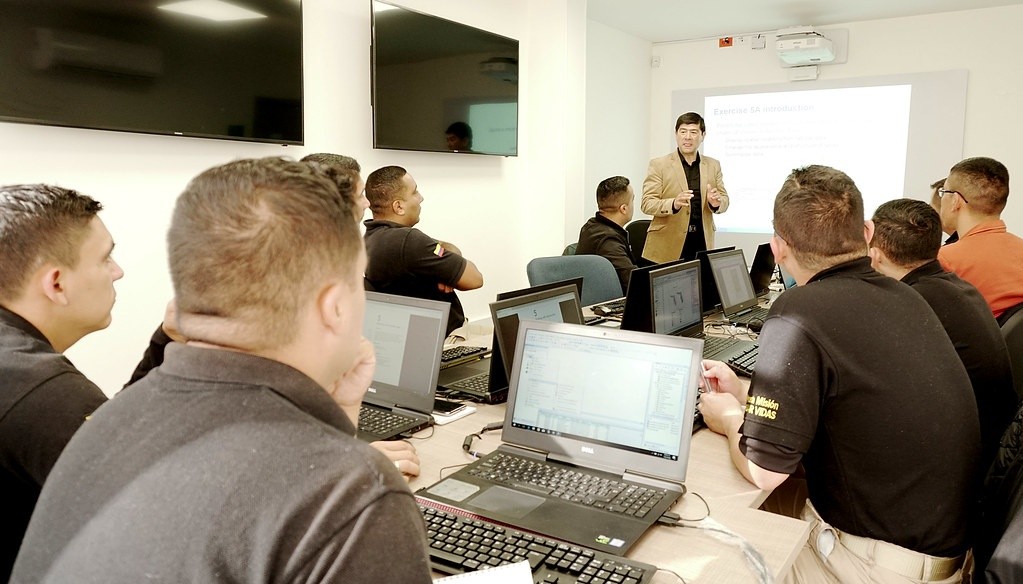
[379,282,820,584]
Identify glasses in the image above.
[938,187,968,204]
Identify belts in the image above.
[839,531,967,581]
[687,224,703,232]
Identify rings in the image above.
[395,461,399,470]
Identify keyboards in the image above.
[440,344,492,371]
[723,343,759,377]
[583,316,607,327]
[591,298,627,314]
[692,387,705,434]
[416,504,658,584]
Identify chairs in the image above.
[526,253,624,306]
[944,311,1023,584]
[625,219,651,265]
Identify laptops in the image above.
[356,243,797,557]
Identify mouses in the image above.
[594,306,612,317]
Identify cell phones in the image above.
[431,399,466,417]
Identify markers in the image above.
[769,287,782,292]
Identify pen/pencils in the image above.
[701,363,712,392]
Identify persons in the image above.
[300,153,370,226]
[869,198,1017,471]
[0,184,421,584]
[938,158,1023,320]
[364,166,483,337]
[641,112,729,267]
[7,159,432,583]
[445,122,472,150]
[697,166,986,583]
[930,178,959,245]
[574,176,637,295]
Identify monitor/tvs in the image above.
[0,0,305,145]
[370,0,519,157]
